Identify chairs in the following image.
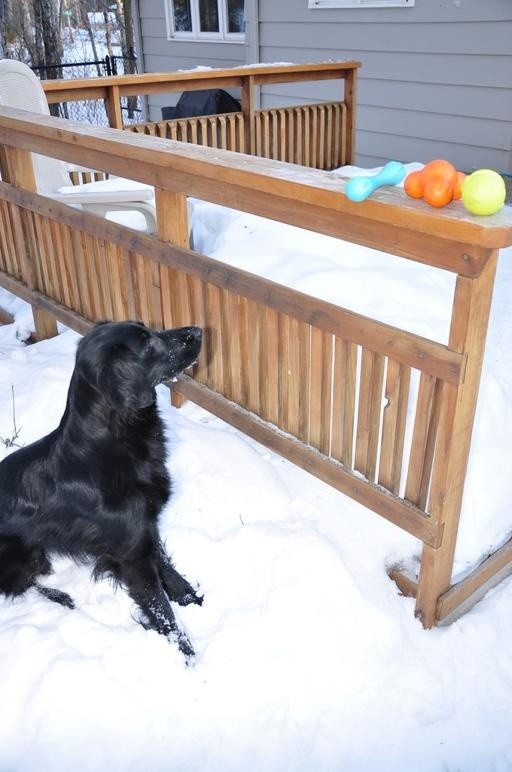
[0,56,202,254]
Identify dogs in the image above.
[0,320,203,668]
